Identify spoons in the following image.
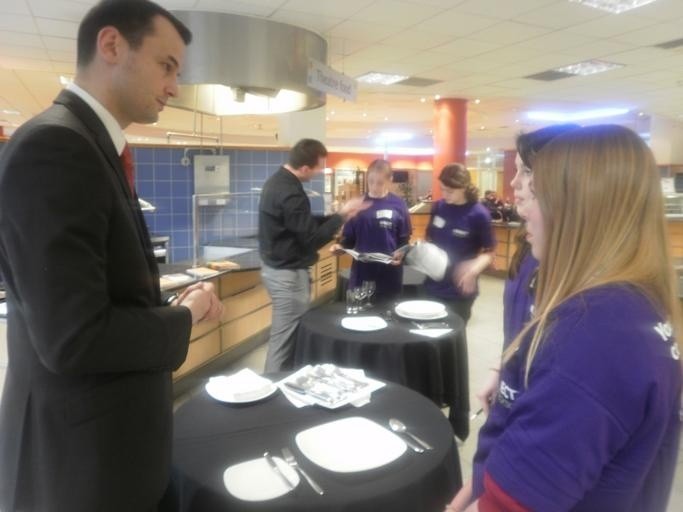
[388,417,434,451]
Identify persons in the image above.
[418,162,498,326]
[471,125,576,419]
[442,124,682,512]
[333,160,412,300]
[256,136,375,374]
[420,186,521,224]
[0,2,226,510]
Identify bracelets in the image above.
[487,367,502,375]
[444,501,455,510]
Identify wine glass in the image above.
[355,280,374,311]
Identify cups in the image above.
[345,289,357,315]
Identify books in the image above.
[333,247,394,265]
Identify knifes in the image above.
[261,449,295,492]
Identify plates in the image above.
[221,457,299,503]
[340,316,386,332]
[205,373,276,404]
[293,415,406,475]
[392,299,449,320]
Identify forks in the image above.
[282,446,326,498]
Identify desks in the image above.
[169,363,463,512]
[291,299,468,440]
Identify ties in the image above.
[121,142,135,200]
